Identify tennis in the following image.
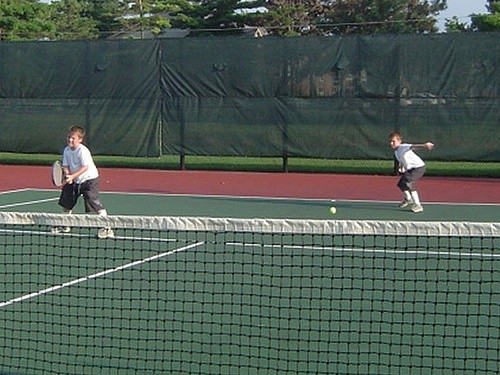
[329,207,337,214]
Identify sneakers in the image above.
[412,204,423,213]
[98,228,115,238]
[50,227,70,233]
[399,198,414,208]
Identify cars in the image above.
[387,91,445,107]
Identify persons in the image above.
[389,131,434,213]
[51,125,115,239]
